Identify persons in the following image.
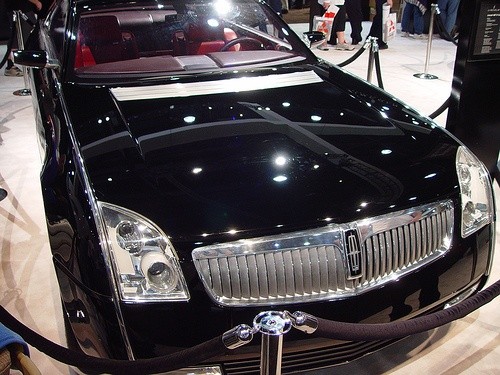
[4,0,42,77]
[308,0,458,51]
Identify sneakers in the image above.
[317,45,329,51]
[5,66,23,76]
[414,34,428,39]
[337,43,355,50]
[400,32,409,39]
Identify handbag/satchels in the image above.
[383,13,396,43]
[312,7,334,43]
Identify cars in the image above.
[13,0,500,375]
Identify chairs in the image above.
[75,14,126,66]
[177,14,239,53]
